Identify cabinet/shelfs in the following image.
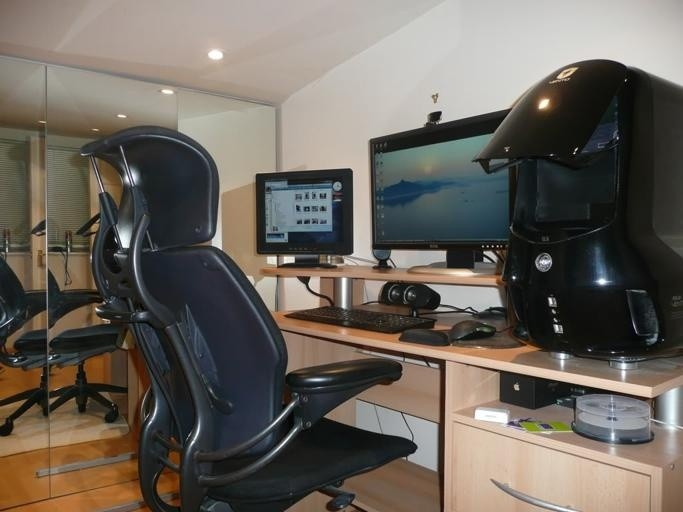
[258,259,681,511]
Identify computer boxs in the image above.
[473,59,683,370]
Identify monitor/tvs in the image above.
[369,107,518,277]
[256,168,353,268]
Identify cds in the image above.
[576,411,649,438]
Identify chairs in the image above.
[0,253,127,435]
[78,124,418,511]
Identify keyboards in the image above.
[284,306,435,334]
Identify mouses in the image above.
[449,320,496,340]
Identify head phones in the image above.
[380,282,441,309]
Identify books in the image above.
[518,419,574,434]
